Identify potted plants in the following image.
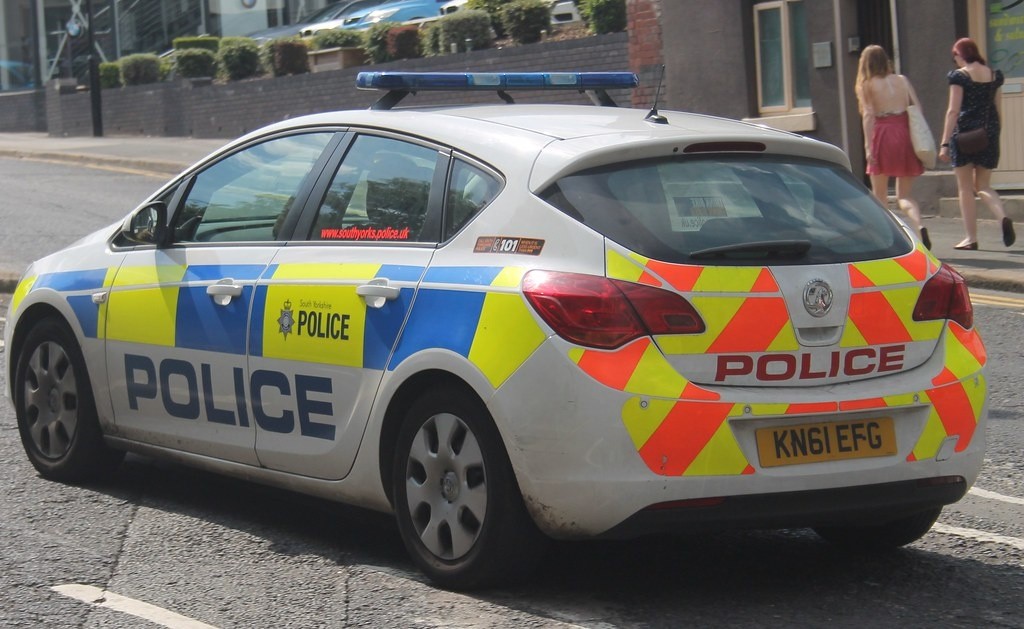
[305,28,365,71]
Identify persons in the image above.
[853,44,932,251]
[938,38,1016,251]
[349,155,418,230]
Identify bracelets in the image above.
[941,143,949,147]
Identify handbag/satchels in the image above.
[957,128,988,154]
[906,104,937,169]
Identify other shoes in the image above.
[953,239,978,250]
[1001,217,1016,247]
[918,226,931,252]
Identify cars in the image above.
[0,72,992,595]
[0,59,39,92]
[239,0,588,42]
